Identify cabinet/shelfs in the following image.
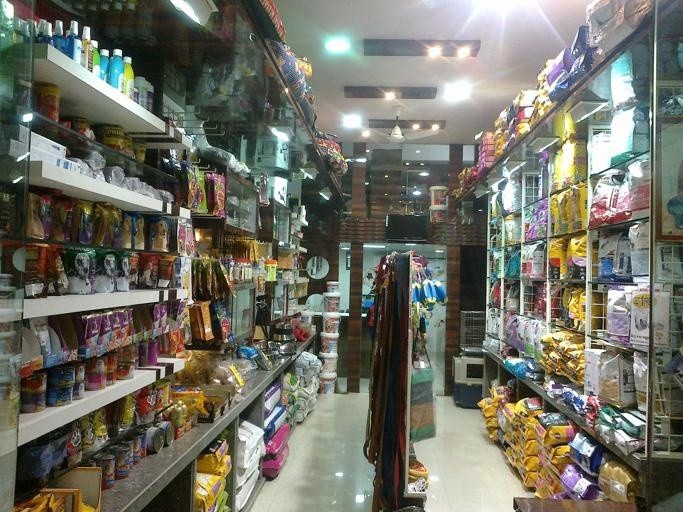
[446,0,682,509]
[0,0,349,508]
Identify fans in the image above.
[363,124,438,143]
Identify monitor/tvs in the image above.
[385,213,429,242]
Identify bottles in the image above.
[109,49,124,96]
[90,40,100,78]
[52,20,64,54]
[99,48,109,83]
[122,56,134,100]
[64,20,82,64]
[81,25,93,72]
[39,22,53,46]
[36,18,47,43]
